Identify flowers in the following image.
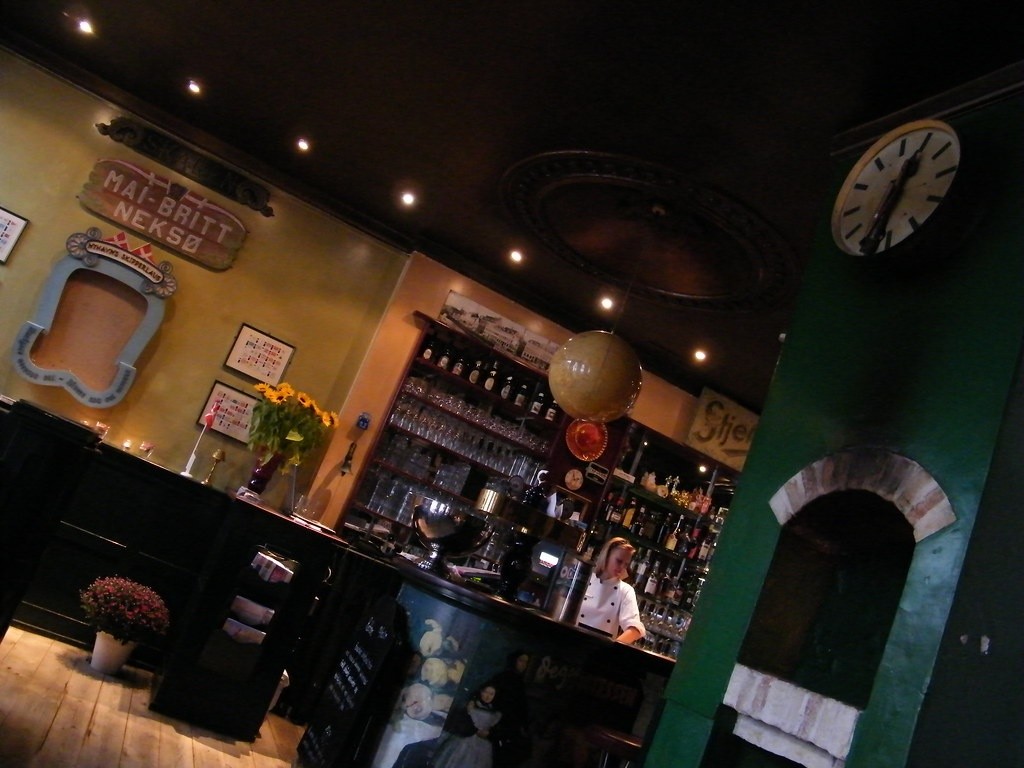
[80,576,171,645]
[250,379,338,476]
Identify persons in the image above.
[576,537,646,644]
[430,649,532,768]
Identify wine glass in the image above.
[388,370,555,487]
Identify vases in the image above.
[91,629,139,674]
[249,439,290,493]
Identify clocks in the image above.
[830,115,981,267]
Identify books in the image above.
[223,617,266,645]
[231,594,275,624]
[252,552,295,582]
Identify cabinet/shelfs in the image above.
[341,308,741,655]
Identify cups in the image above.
[349,432,471,532]
[95,420,111,443]
[138,439,156,459]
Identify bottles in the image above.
[575,471,729,660]
[418,341,560,424]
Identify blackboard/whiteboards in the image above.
[295,631,398,768]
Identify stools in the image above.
[587,724,647,768]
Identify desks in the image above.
[1,398,347,672]
